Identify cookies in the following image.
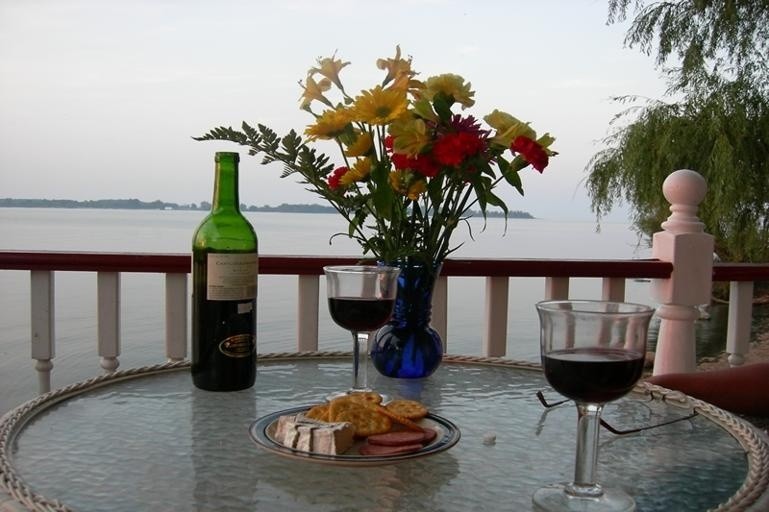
[306,390,437,457]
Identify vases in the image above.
[370,259,443,378]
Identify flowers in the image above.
[190,43,561,373]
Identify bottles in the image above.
[189,151,260,394]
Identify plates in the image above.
[248,403,463,467]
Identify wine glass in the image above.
[323,263,402,406]
[532,298,658,511]
